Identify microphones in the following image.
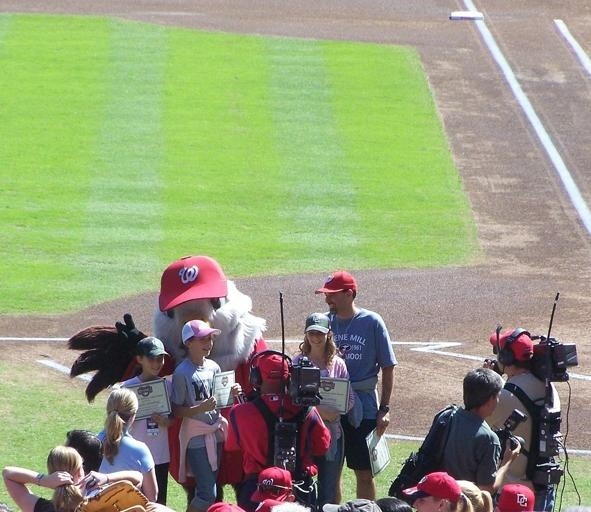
[496,326,502,352]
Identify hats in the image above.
[182,320,223,346]
[491,328,535,361]
[499,483,536,511]
[137,337,171,358]
[251,466,293,502]
[207,498,383,512]
[403,471,461,502]
[305,312,331,334]
[315,270,357,294]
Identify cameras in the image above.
[492,408,528,460]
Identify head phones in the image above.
[498,327,531,366]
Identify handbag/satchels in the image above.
[389,408,454,496]
